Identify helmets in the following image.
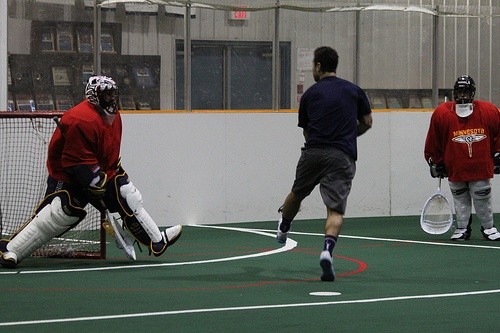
[84,75,118,114]
[453,75,476,103]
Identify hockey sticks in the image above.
[421,96,454,235]
[54,116,137,262]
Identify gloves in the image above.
[428,159,447,179]
[493,153,500,174]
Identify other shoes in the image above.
[0,251,18,269]
[152,224,182,256]
[481,226,500,241]
[451,228,472,241]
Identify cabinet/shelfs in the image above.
[9,20,161,112]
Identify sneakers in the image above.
[276,217,292,244]
[319,250,335,282]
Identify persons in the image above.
[423,74,500,241]
[0,74,183,268]
[277,46,372,283]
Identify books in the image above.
[137,88,153,109]
[76,61,94,84]
[54,88,74,111]
[118,89,135,111]
[72,86,85,106]
[4,92,15,112]
[7,59,53,90]
[76,24,93,53]
[49,64,69,87]
[130,62,154,89]
[100,63,132,86]
[55,23,74,51]
[33,91,54,111]
[15,91,35,110]
[101,27,115,53]
[39,26,55,51]
